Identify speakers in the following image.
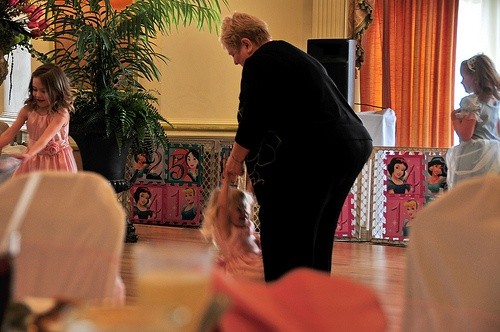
[307,38,356,110]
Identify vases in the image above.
[0,51,9,85]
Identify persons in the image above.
[219,13,373,283]
[200,167,264,283]
[0,62,78,179]
[448,53,500,187]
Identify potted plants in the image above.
[21,0,234,243]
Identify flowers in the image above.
[0,0,51,104]
